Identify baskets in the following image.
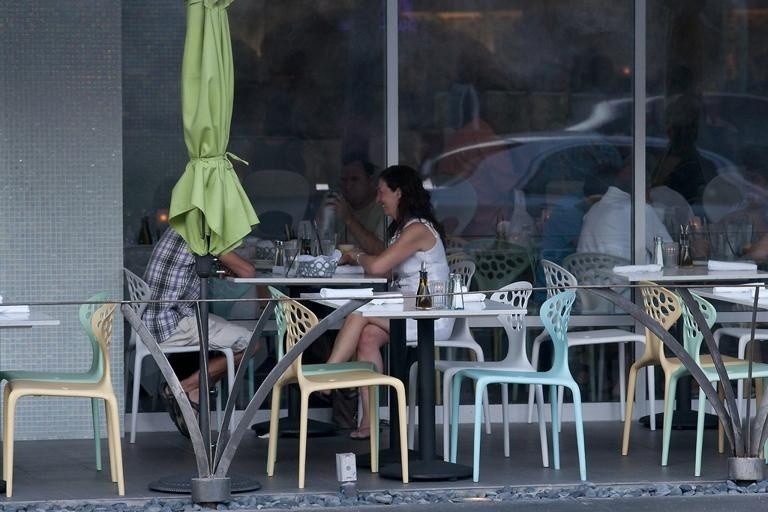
[298,259,339,278]
[255,246,276,260]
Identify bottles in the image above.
[653,224,694,268]
[415,260,465,310]
[138,216,153,245]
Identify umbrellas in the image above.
[169,1,260,470]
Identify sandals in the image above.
[161,381,199,439]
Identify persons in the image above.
[136,207,268,443]
[310,154,386,261]
[438,91,525,246]
[526,157,768,395]
[298,165,451,440]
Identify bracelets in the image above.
[356,251,366,265]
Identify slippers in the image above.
[314,391,330,402]
[350,427,383,441]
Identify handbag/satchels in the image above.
[332,389,357,426]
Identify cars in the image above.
[421,134,768,268]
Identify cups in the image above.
[275,231,355,277]
[704,223,752,262]
